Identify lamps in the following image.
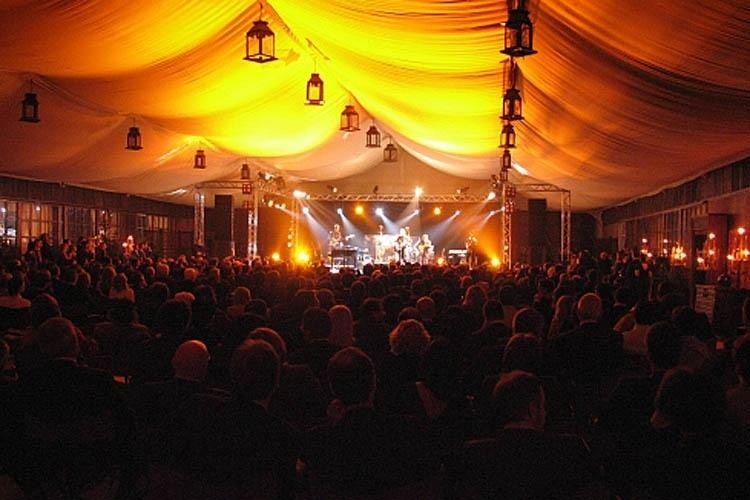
[18,80,42,124]
[242,2,279,64]
[124,116,144,151]
[303,57,326,106]
[339,94,361,133]
[500,1,538,58]
[239,164,520,210]
[383,135,399,162]
[365,117,382,148]
[500,147,513,169]
[500,120,517,149]
[193,141,206,170]
[499,70,525,120]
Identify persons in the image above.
[0,221,750,500]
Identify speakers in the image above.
[213,194,234,257]
[526,199,547,266]
